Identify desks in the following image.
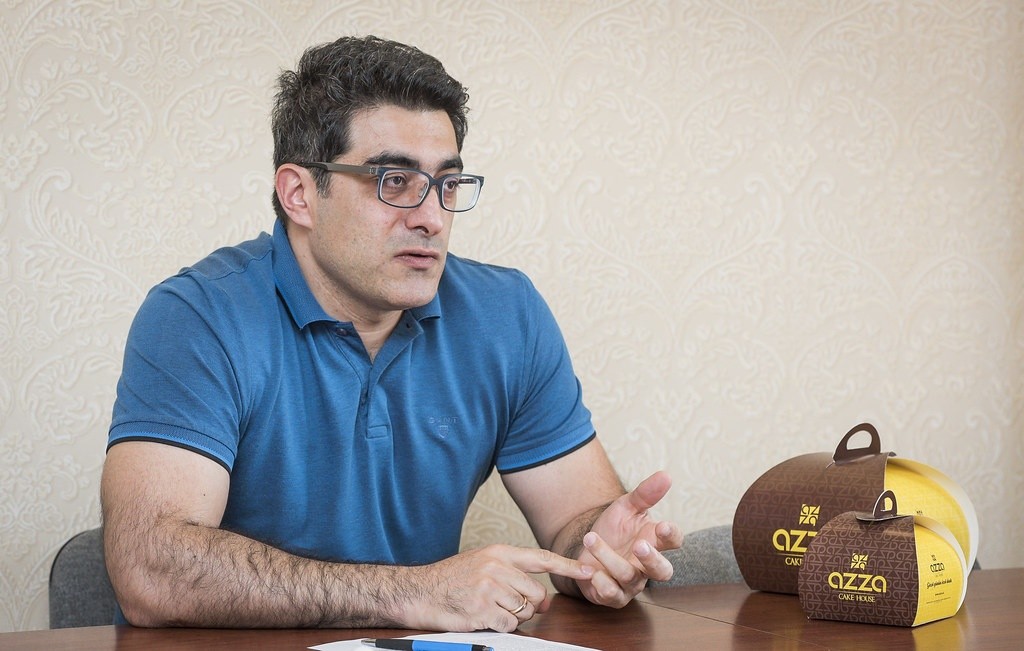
[0,564,1024,651]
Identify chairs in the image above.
[48,526,117,629]
[643,523,742,588]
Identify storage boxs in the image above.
[732,423,980,596]
[800,490,969,631]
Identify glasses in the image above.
[300,162,484,212]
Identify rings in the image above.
[511,593,528,613]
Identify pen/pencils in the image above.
[361,637,494,651]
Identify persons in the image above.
[99,37,682,631]
